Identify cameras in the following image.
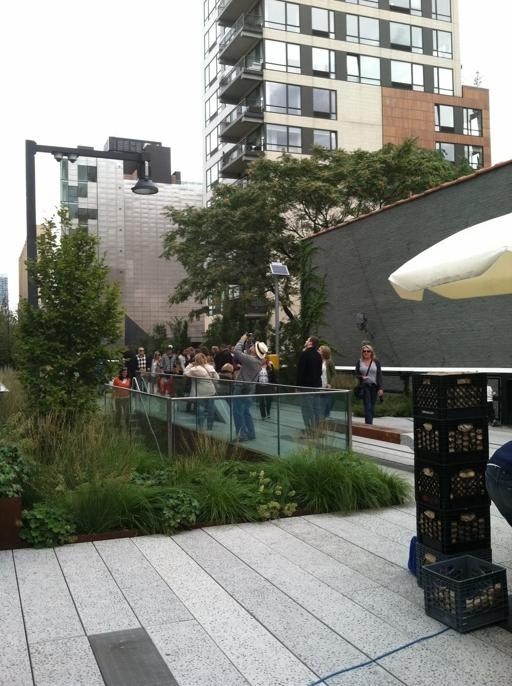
[246,332,252,339]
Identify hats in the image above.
[255,341,269,359]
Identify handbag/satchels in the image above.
[353,381,366,400]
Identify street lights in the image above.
[21,134,161,313]
[268,261,292,371]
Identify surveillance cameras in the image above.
[54,152,63,162]
[68,154,78,163]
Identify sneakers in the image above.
[232,416,270,442]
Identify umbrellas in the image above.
[388,212,512,302]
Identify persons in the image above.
[352,345,384,425]
[485,440,512,528]
[294,336,323,439]
[317,345,336,420]
[111,331,277,442]
